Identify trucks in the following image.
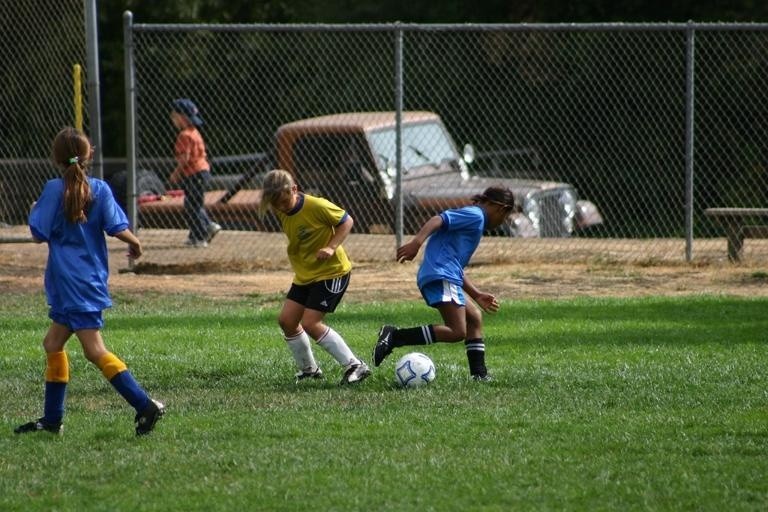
[106,107,611,239]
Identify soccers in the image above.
[394,352,437,387]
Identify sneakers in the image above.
[134,400,166,435]
[340,360,373,387]
[207,222,221,242]
[14,418,64,435]
[374,326,396,366]
[182,239,208,247]
[293,366,322,377]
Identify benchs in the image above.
[702,206,767,262]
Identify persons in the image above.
[166,95,222,248]
[260,167,370,388]
[12,126,167,438]
[372,183,518,383]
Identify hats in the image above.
[170,100,203,125]
